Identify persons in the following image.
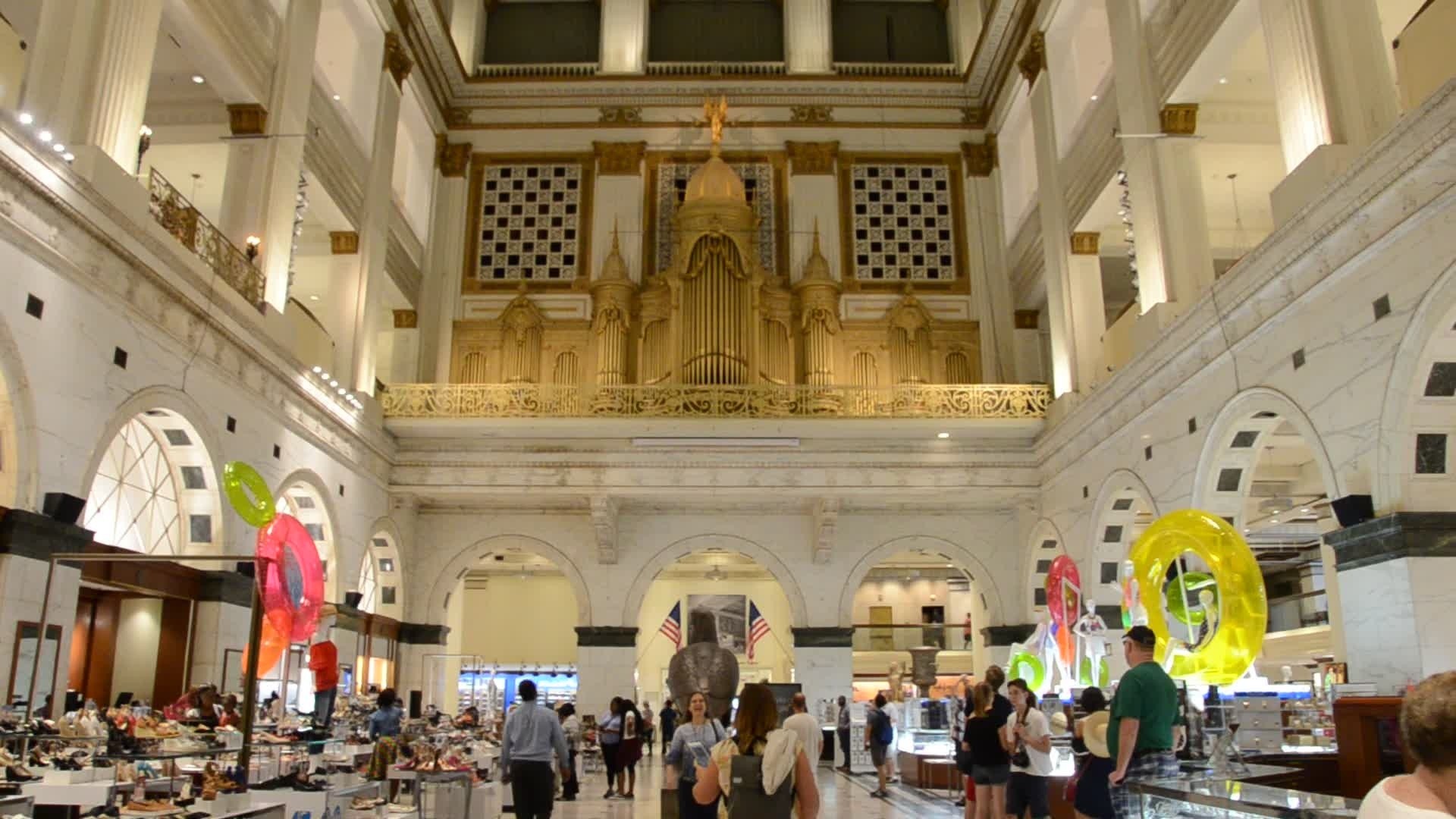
[1073,600,1109,687]
[1161,590,1219,674]
[0,624,1178,819]
[485,668,499,733]
[307,603,338,785]
[1120,559,1149,628]
[1355,669,1456,819]
[1038,621,1072,700]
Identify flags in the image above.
[660,600,684,652]
[746,600,770,660]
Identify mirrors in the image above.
[8,621,63,753]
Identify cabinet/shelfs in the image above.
[1235,695,1284,748]
[0,704,601,819]
[1245,758,1342,792]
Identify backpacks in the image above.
[872,708,893,744]
[724,737,795,819]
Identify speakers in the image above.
[1331,495,1374,527]
[237,562,255,578]
[44,492,86,524]
[347,592,363,608]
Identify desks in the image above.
[923,758,962,800]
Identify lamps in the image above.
[1256,495,1294,516]
[244,236,259,261]
[135,124,153,181]
[705,565,731,582]
[513,565,533,581]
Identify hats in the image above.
[1121,625,1155,645]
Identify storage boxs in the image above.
[1287,734,1331,747]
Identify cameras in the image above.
[1012,752,1029,768]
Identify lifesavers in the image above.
[1044,553,1081,627]
[224,460,274,529]
[1166,572,1219,625]
[1009,651,1046,692]
[254,515,324,642]
[1130,508,1268,687]
[1081,655,1110,689]
[242,612,289,680]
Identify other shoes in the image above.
[884,775,898,783]
[956,799,964,806]
[604,790,613,799]
[870,790,886,797]
[556,795,575,801]
[1,730,485,819]
